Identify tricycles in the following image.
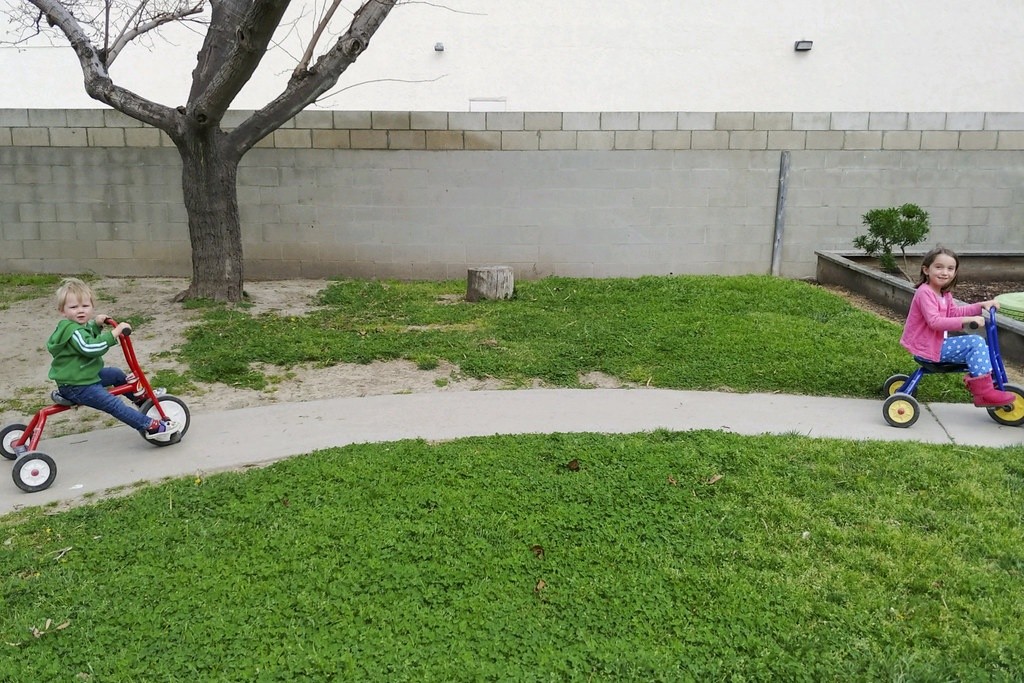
[882,306,1024,428]
[0,317,191,494]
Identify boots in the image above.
[963,373,1016,407]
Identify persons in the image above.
[899,247,1016,408]
[45,276,181,439]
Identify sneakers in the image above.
[145,420,181,439]
[134,387,167,410]
